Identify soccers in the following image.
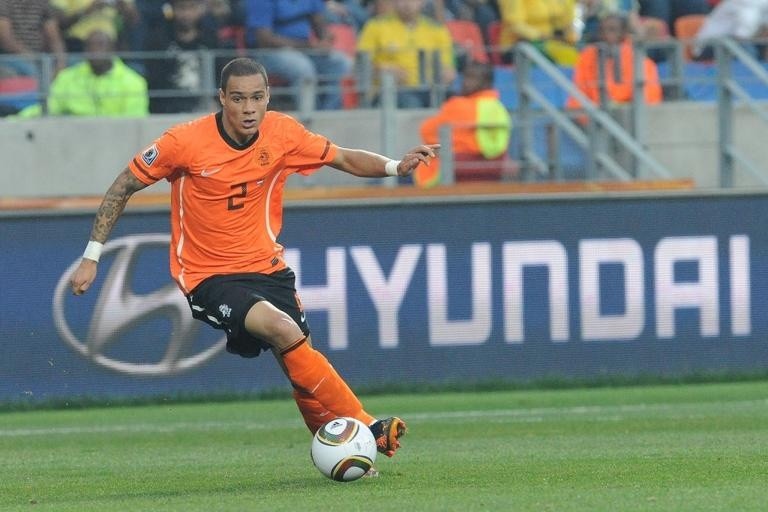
[310,417,377,482]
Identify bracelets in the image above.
[82,240,105,263]
[385,159,402,177]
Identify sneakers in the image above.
[363,416,407,479]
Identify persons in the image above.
[357,1,457,109]
[69,54,442,477]
[424,1,768,63]
[398,57,508,185]
[569,7,662,125]
[244,0,371,113]
[0,1,236,75]
[48,14,150,116]
